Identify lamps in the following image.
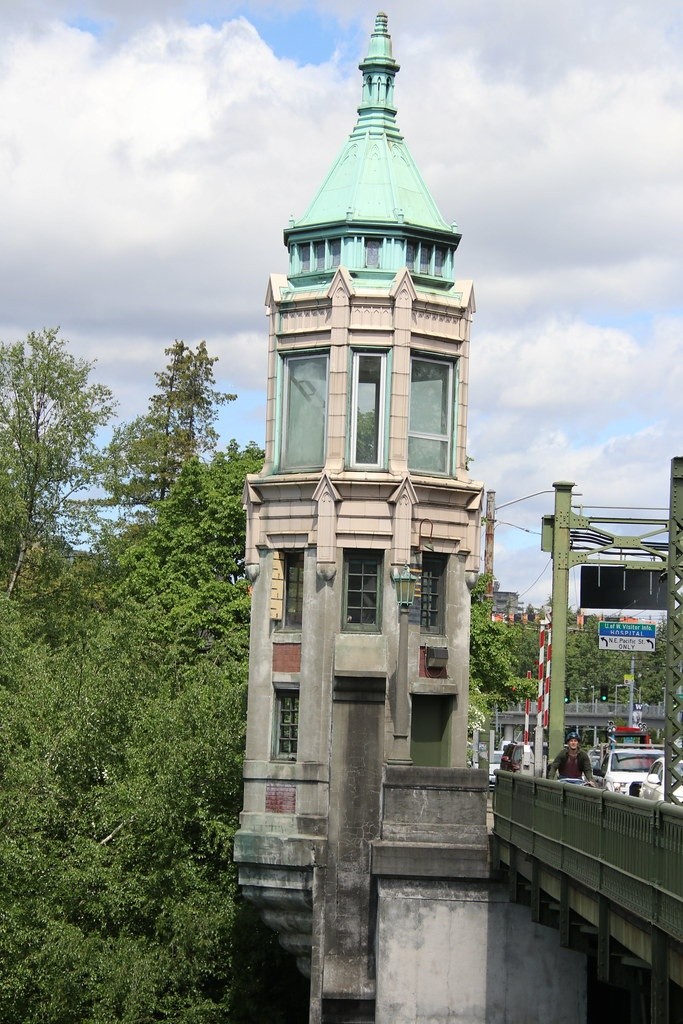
[426,646,448,670]
[415,518,435,555]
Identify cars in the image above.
[467,751,504,787]
[498,740,600,781]
[638,757,683,805]
[592,749,666,796]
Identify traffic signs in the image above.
[598,621,655,651]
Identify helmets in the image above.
[566,731,581,742]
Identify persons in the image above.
[547,732,598,788]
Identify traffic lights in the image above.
[565,687,570,704]
[601,685,608,703]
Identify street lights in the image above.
[616,685,641,704]
[484,490,583,620]
